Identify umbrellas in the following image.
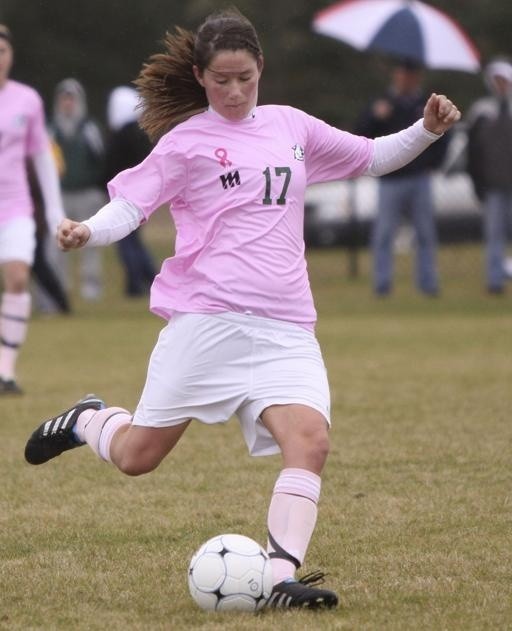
[310,0,483,75]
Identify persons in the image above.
[25,7,461,614]
[95,86,159,297]
[0,22,65,396]
[351,60,453,298]
[465,59,512,293]
[35,76,107,313]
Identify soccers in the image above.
[187,534,269,614]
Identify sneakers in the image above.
[264,572,337,613]
[24,393,105,466]
